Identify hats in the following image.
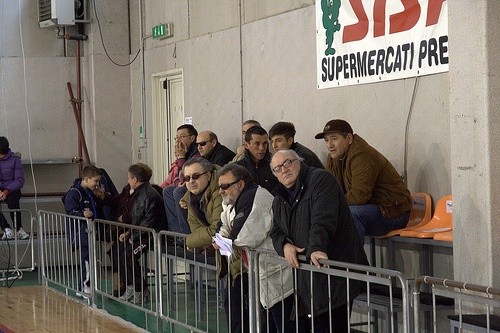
[315,119,353,139]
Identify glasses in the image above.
[174,135,191,140]
[194,140,212,147]
[273,158,298,173]
[217,180,238,191]
[185,172,207,181]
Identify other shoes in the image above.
[76,290,90,299]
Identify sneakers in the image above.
[2,228,14,239]
[18,228,29,239]
[120,286,134,300]
[131,289,149,303]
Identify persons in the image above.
[63,164,119,300]
[269,148,373,333]
[210,163,294,333]
[0,137,30,240]
[96,163,170,304]
[315,119,412,251]
[156,119,342,259]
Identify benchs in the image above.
[367,192,453,296]
[109,237,500,333]
[0,192,66,203]
